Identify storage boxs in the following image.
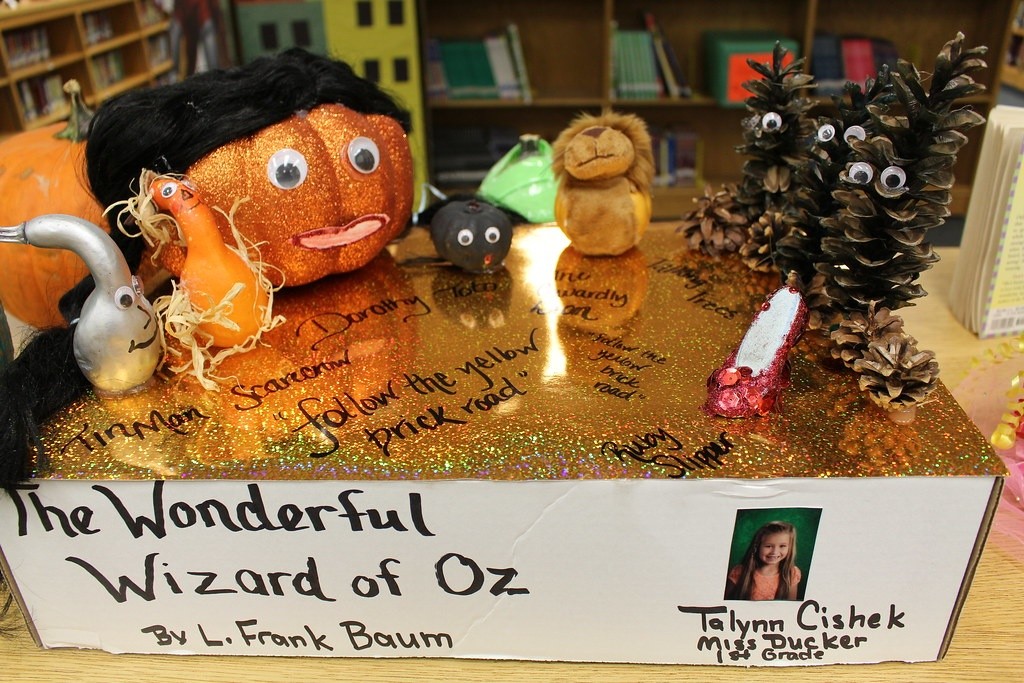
[2,236,1007,668]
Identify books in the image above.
[613,11,694,100]
[649,124,705,190]
[425,22,533,102]
[2,0,175,122]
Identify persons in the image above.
[723,520,802,601]
[165,2,222,82]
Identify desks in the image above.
[0,245,1024,681]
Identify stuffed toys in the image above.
[552,108,658,256]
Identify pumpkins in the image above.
[430,200,514,273]
[0,78,110,330]
[152,103,414,286]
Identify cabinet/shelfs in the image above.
[0,1,194,128]
[419,0,1024,222]
